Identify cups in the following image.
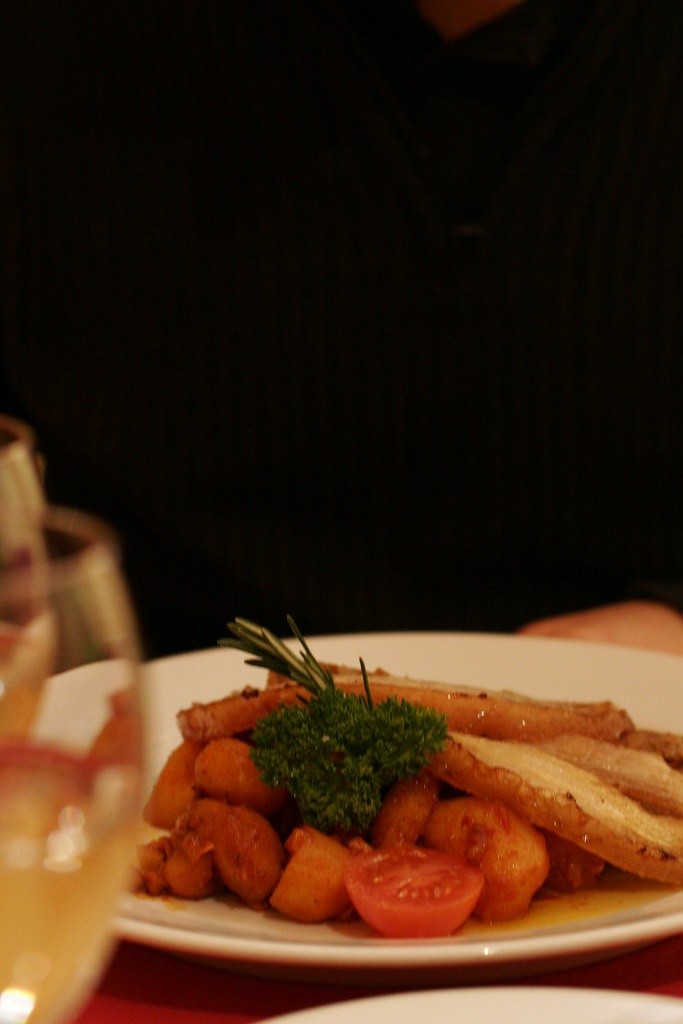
[0,507,151,1024]
[0,414,57,750]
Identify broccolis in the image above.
[216,613,452,842]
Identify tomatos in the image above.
[345,844,485,937]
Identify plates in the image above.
[259,983,683,1024]
[38,630,683,967]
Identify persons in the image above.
[0,0,683,659]
